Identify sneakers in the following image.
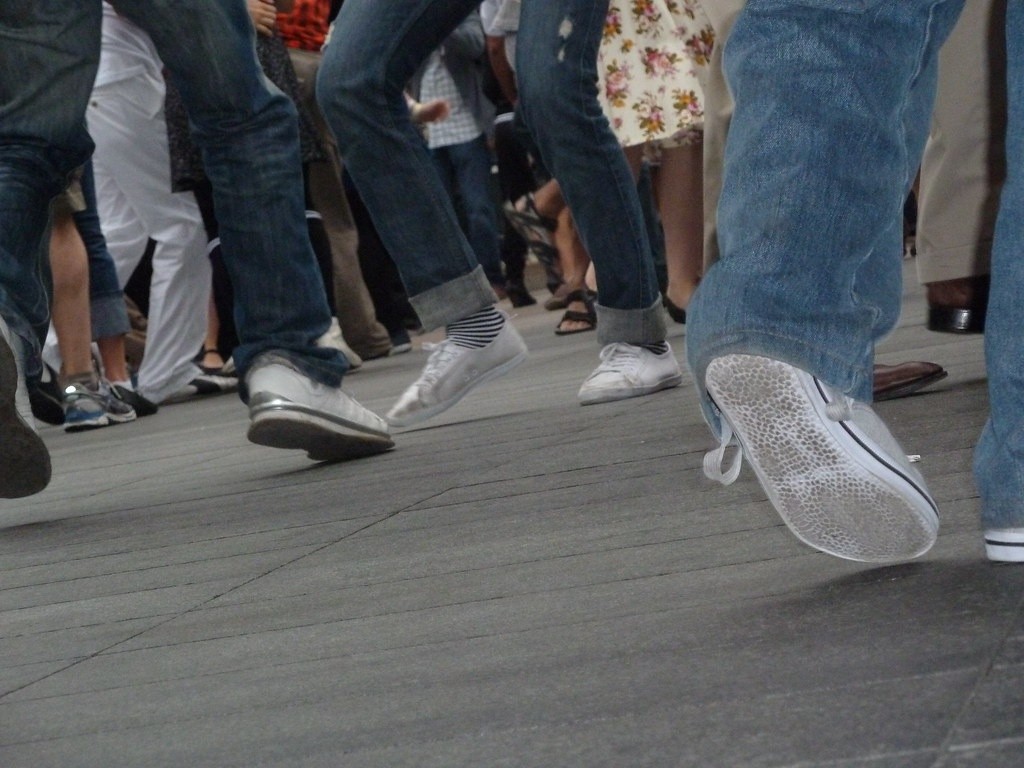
[983,527,1024,562]
[383,308,530,428]
[0,316,52,499]
[55,375,137,433]
[576,341,683,406]
[701,353,942,567]
[243,352,395,459]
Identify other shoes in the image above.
[585,288,599,296]
[544,282,589,310]
[312,316,364,374]
[661,293,686,324]
[872,360,948,401]
[28,358,67,426]
[218,355,236,375]
[547,276,561,295]
[196,348,224,376]
[924,276,989,333]
[108,385,158,417]
[386,328,413,356]
[164,374,238,402]
[505,281,537,307]
[204,208,323,270]
[901,243,914,258]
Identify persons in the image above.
[685,0,1024,564]
[35,0,1006,434]
[317,0,680,427]
[0,0,396,500]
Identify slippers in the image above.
[554,287,598,334]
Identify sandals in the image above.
[500,188,564,269]
